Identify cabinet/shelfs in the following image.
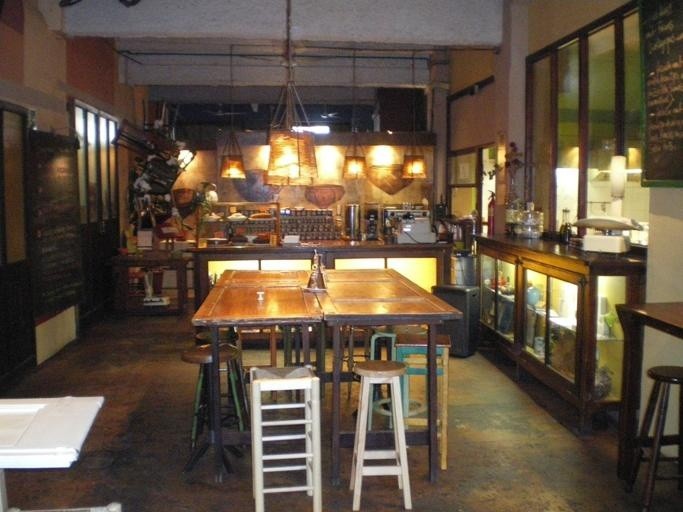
[197,202,282,248]
[472,231,647,439]
[115,253,189,316]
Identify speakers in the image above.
[431,284,482,359]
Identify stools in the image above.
[196,330,243,436]
[626,366,683,512]
[348,360,412,511]
[181,342,243,451]
[367,326,429,431]
[395,333,452,471]
[248,366,321,512]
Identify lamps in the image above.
[219,44,247,180]
[268,1,319,179]
[401,54,428,179]
[342,48,368,180]
[265,40,314,187]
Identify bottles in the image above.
[560,209,573,246]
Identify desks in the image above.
[615,303,683,494]
[307,268,464,487]
[1,396,102,508]
[192,268,324,489]
